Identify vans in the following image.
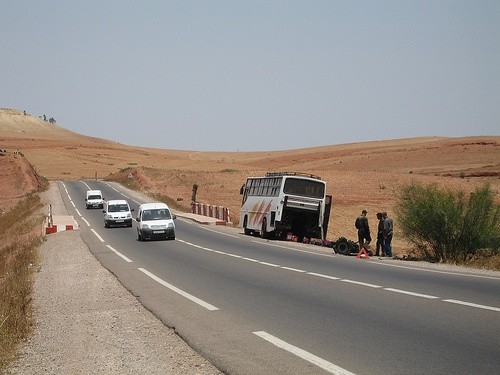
[136,202,177,242]
[102,200,135,229]
[85,189,105,210]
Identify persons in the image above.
[382,212,394,257]
[373,213,386,256]
[355,210,372,252]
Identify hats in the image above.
[362,210,367,213]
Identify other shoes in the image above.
[374,253,386,256]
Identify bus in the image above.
[239,171,332,243]
